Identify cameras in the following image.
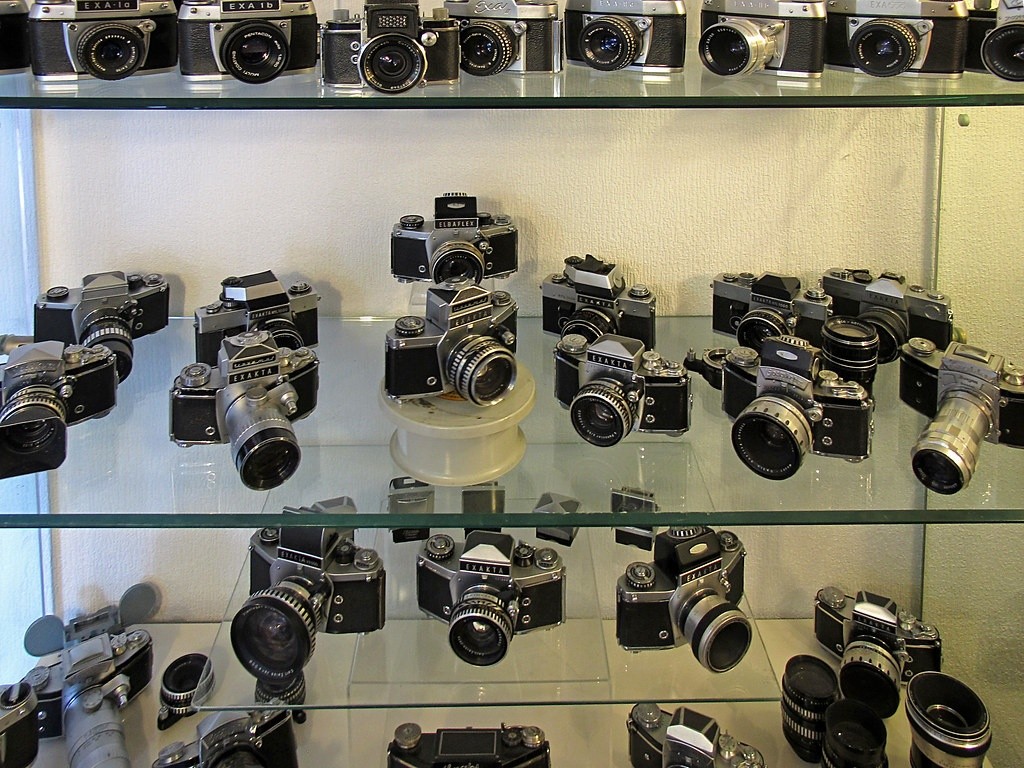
[0,0,1024,93]
[0,192,1024,768]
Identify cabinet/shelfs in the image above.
[1,66,1024,768]
